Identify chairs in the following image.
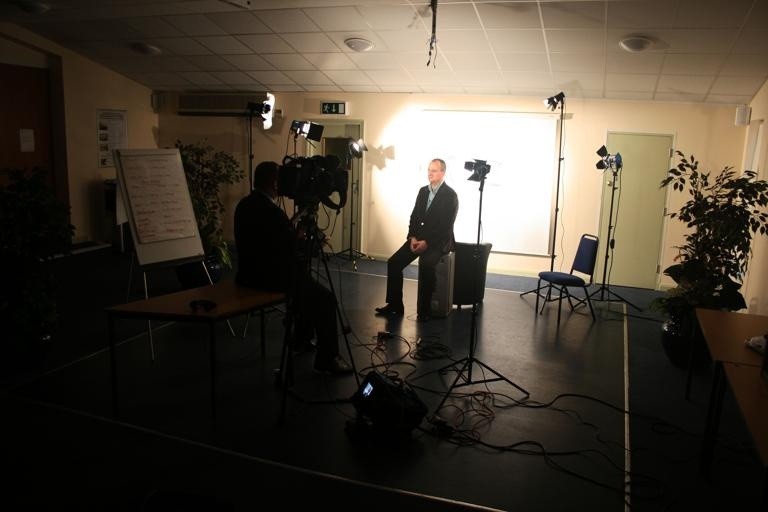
[532,233,601,330]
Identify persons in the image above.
[234,161,353,377]
[376,158,459,323]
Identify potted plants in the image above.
[647,146,768,371]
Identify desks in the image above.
[693,304,768,512]
[100,279,286,429]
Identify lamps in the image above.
[343,34,376,55]
[619,36,655,54]
[290,118,325,229]
[320,136,376,271]
[574,144,645,313]
[517,88,588,318]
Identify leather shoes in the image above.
[417,311,429,321]
[376,303,403,315]
[305,338,321,353]
[313,359,352,376]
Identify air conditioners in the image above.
[175,91,269,119]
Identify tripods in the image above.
[404,247,531,422]
[521,118,588,315]
[276,213,365,392]
[572,183,646,316]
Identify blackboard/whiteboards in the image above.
[111,147,206,271]
